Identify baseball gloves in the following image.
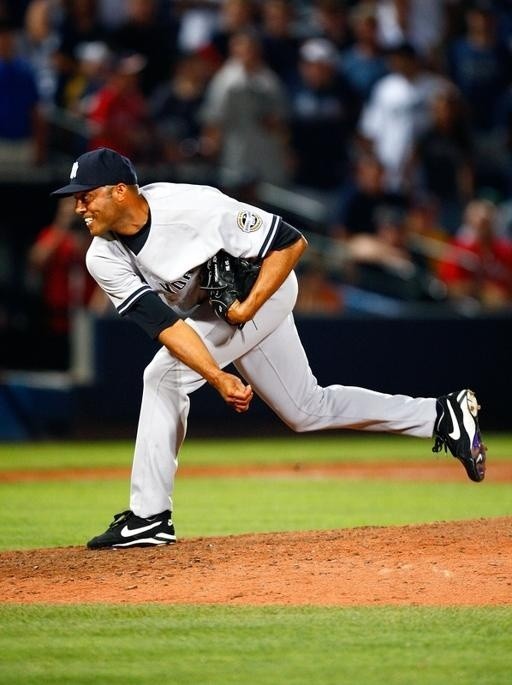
[199,250,263,325]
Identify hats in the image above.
[50,146,137,199]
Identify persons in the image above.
[70,148,486,551]
[1,0,511,365]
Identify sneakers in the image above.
[435,389,487,482]
[87,509,177,549]
